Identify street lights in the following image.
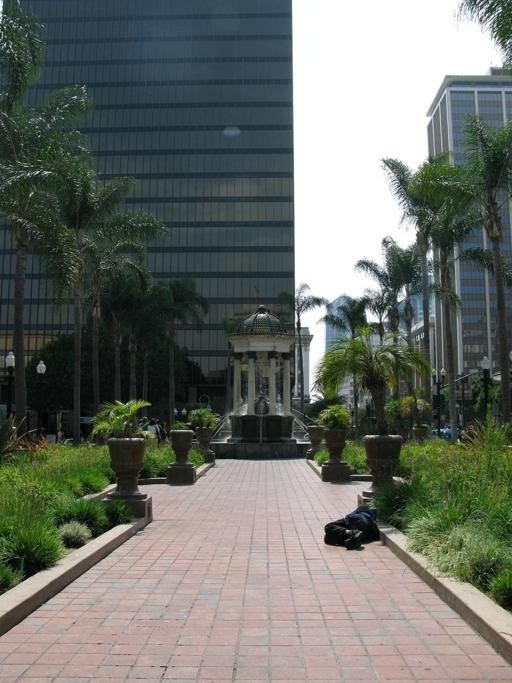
[481,355,491,423]
[349,405,355,440]
[6,351,16,415]
[35,359,47,440]
[432,367,447,438]
[174,406,187,422]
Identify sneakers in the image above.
[345,529,363,549]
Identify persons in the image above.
[325,504,381,554]
[431,424,469,444]
[140,416,169,441]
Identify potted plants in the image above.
[306,325,434,496]
[86,397,156,499]
[168,408,220,464]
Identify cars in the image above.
[63,437,84,445]
